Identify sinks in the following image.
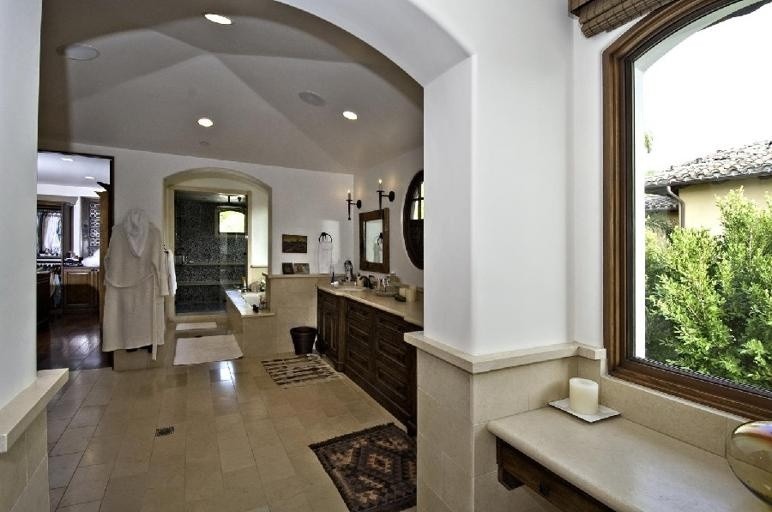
[338,287,360,291]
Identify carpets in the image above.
[176,322,217,330]
[260,354,343,392]
[173,334,243,366]
[307,422,417,512]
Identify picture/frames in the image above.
[359,208,389,273]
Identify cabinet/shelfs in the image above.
[63,269,99,308]
[343,298,423,437]
[317,289,343,373]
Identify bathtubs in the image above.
[240,291,265,308]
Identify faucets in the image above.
[359,275,373,288]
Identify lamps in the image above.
[377,179,395,211]
[345,189,361,220]
